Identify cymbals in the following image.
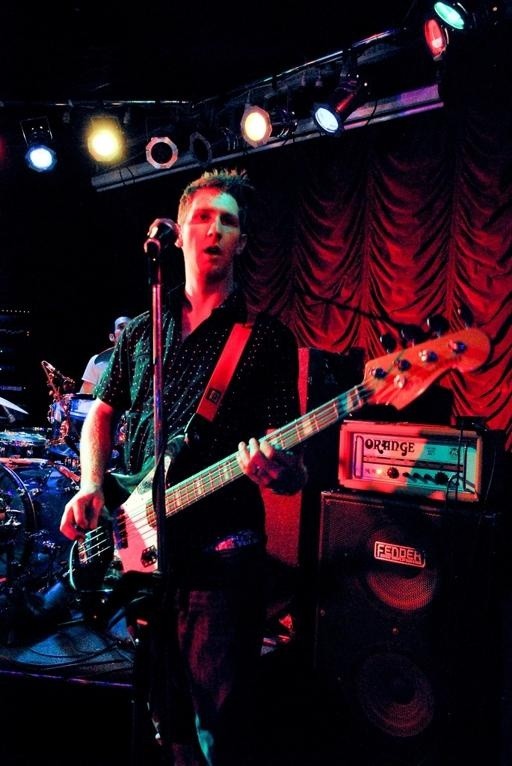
[0,397,29,416]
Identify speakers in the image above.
[317,492,512,766]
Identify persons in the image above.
[81,316,131,393]
[57,171,310,764]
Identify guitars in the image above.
[70,306,493,593]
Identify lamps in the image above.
[312,47,372,138]
[82,105,129,167]
[143,129,180,171]
[420,2,469,64]
[240,103,300,149]
[187,123,243,170]
[19,115,63,174]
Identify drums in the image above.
[45,394,94,457]
[0,456,81,592]
[1,431,46,460]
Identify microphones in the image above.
[46,363,64,381]
[143,218,179,254]
